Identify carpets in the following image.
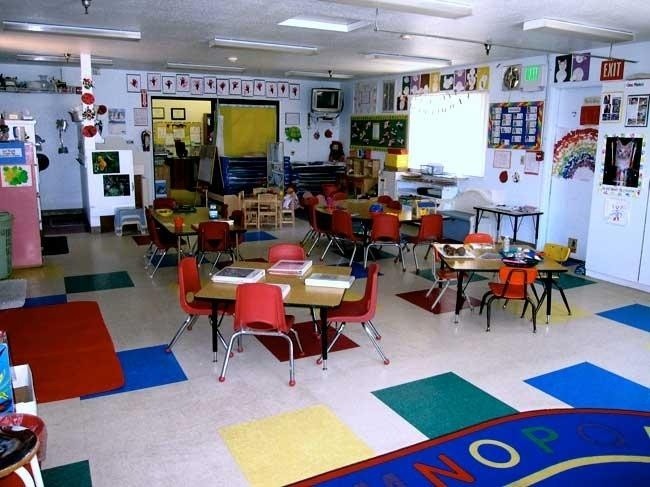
[0,301,125,403]
[282,408,650,487]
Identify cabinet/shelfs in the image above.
[342,156,380,195]
[584,78,650,295]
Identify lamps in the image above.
[285,71,352,79]
[16,53,112,66]
[208,38,317,56]
[324,0,474,20]
[523,19,633,42]
[166,62,245,73]
[358,52,452,67]
[2,20,142,41]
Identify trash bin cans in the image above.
[0,212,15,279]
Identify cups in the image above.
[174,216,183,228]
[16,125,25,141]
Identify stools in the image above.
[114,208,147,236]
[0,426,44,487]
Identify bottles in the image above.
[515,247,524,258]
[48,80,55,90]
[208,203,218,218]
[502,235,510,252]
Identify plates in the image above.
[156,208,172,217]
[434,243,476,260]
[501,257,539,268]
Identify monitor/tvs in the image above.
[310,87,343,113]
